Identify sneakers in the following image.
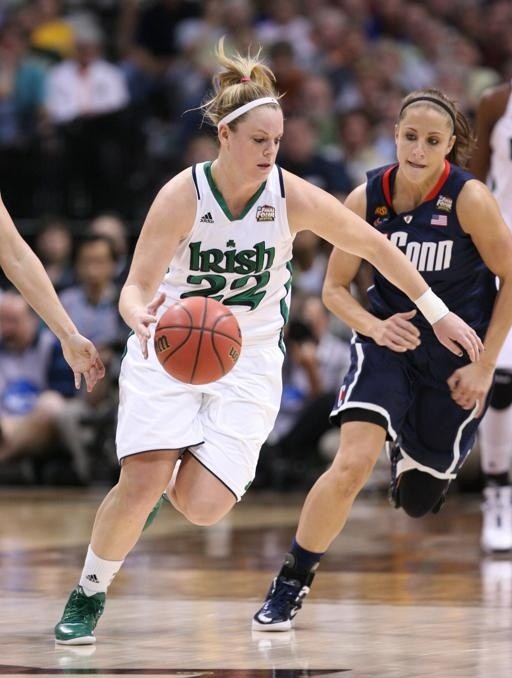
[55,583,105,645]
[478,486,512,557]
[251,551,322,633]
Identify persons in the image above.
[2,193,108,394]
[53,34,489,650]
[254,86,512,634]
[1,1,510,491]
[470,86,510,560]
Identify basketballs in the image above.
[154,296,241,385]
